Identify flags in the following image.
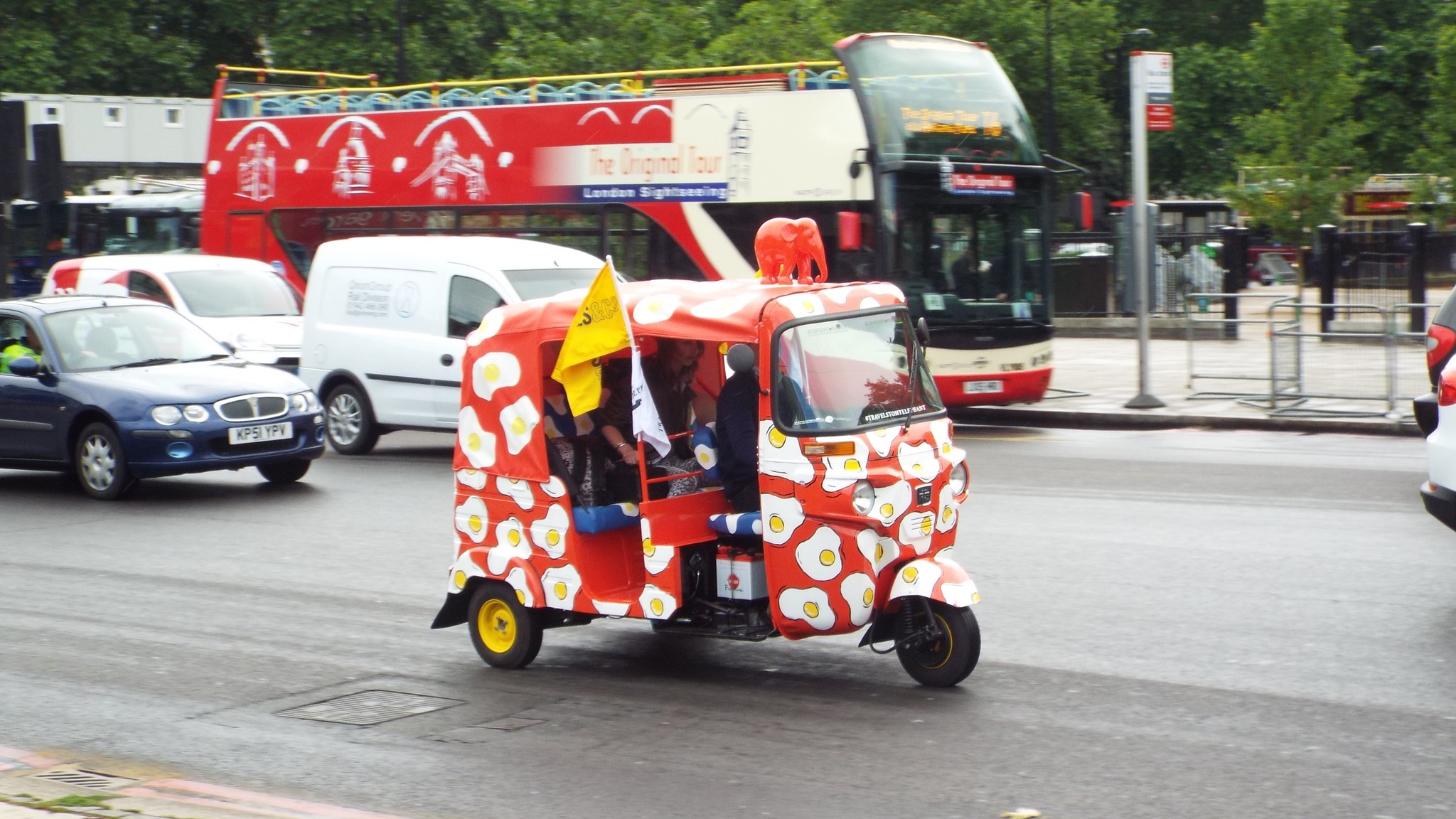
[550,260,635,418]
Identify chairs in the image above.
[692,421,763,534]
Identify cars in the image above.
[0,294,328,500]
[1411,283,1455,533]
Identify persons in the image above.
[2,322,81,375]
[540,337,818,511]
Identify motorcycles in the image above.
[428,213,980,691]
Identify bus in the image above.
[5,175,205,296]
[1341,172,1456,234]
[1228,166,1355,288]
[201,30,1097,410]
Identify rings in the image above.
[627,462,631,464]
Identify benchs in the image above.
[542,394,643,534]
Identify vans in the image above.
[41,251,304,375]
[295,235,631,455]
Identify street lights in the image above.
[1347,45,1387,146]
[1101,28,1157,201]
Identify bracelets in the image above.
[616,441,629,454]
